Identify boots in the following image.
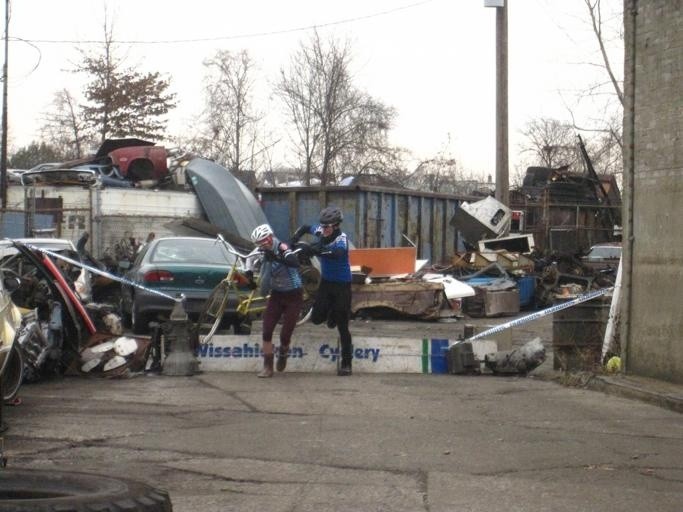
[257,353,274,377]
[276,347,289,372]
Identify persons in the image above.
[241,225,304,379]
[288,204,356,375]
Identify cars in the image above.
[0,237,93,305]
[581,241,622,272]
[118,237,254,336]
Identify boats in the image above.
[183,156,273,251]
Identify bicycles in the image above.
[193,234,321,344]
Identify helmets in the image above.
[319,207,343,226]
[250,224,273,243]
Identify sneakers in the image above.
[328,314,336,328]
[339,362,352,375]
